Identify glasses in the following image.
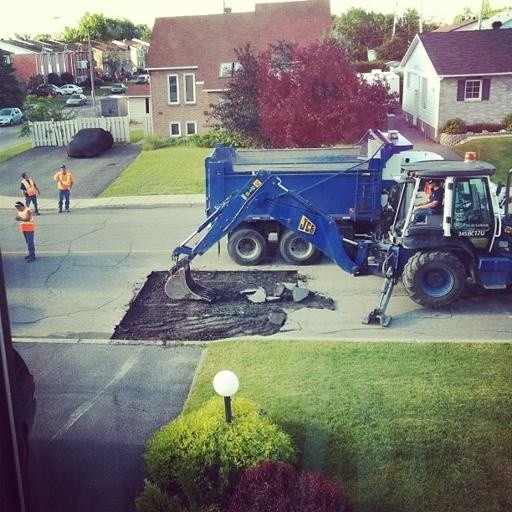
[428,181,434,184]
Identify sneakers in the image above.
[57,208,62,213]
[23,254,36,264]
[65,208,69,212]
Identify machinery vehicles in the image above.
[162,151,512,331]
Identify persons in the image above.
[412,178,445,225]
[14,201,36,263]
[19,172,41,215]
[53,164,74,213]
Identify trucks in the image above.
[203,125,512,280]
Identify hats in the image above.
[59,164,65,168]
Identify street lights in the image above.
[54,14,97,111]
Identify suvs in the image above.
[0,106,24,126]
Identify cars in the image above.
[29,72,149,108]
